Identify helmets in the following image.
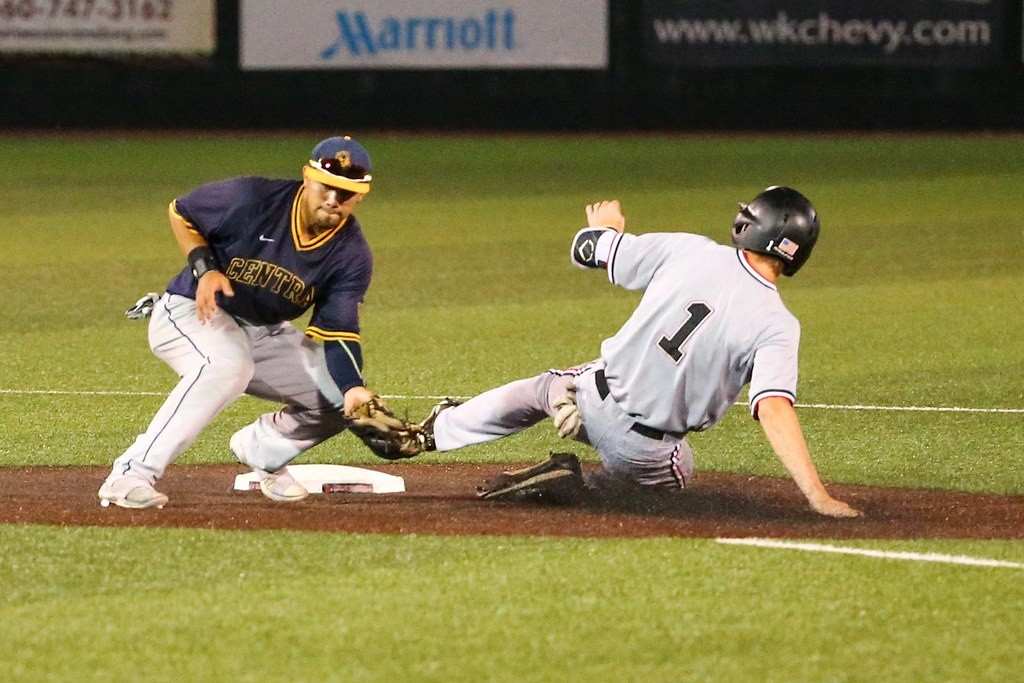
[732,186,821,278]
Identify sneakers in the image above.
[477,451,584,503]
[98,474,168,510]
[420,398,460,451]
[230,432,309,501]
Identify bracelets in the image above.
[187,246,216,278]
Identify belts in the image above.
[595,369,664,441]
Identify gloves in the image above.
[553,381,583,440]
[125,292,162,319]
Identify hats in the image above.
[306,134,372,194]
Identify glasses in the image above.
[310,157,372,182]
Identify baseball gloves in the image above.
[341,394,430,460]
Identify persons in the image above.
[420,186,863,517]
[100,136,431,508]
